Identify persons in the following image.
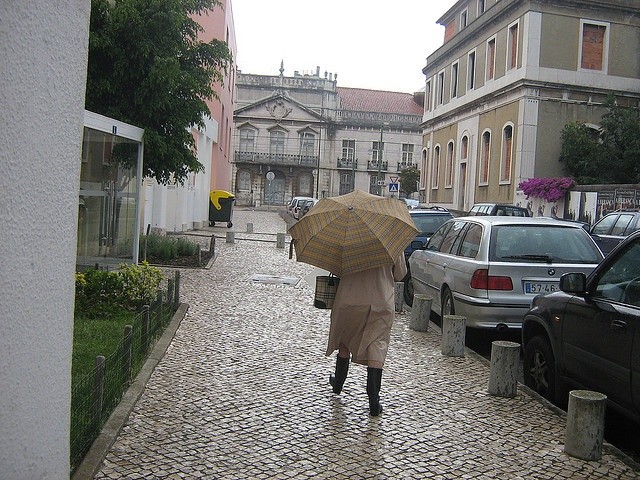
[325,251,408,416]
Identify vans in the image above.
[468,203,530,218]
[587,211,640,253]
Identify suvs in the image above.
[402,205,455,257]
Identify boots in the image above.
[330,353,352,395]
[367,367,382,416]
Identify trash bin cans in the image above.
[209,190,236,228]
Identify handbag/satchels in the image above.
[314,272,340,309]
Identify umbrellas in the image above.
[288,188,422,278]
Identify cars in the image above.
[287,197,307,214]
[291,199,317,218]
[404,217,607,330]
[520,231,639,427]
[298,201,314,218]
